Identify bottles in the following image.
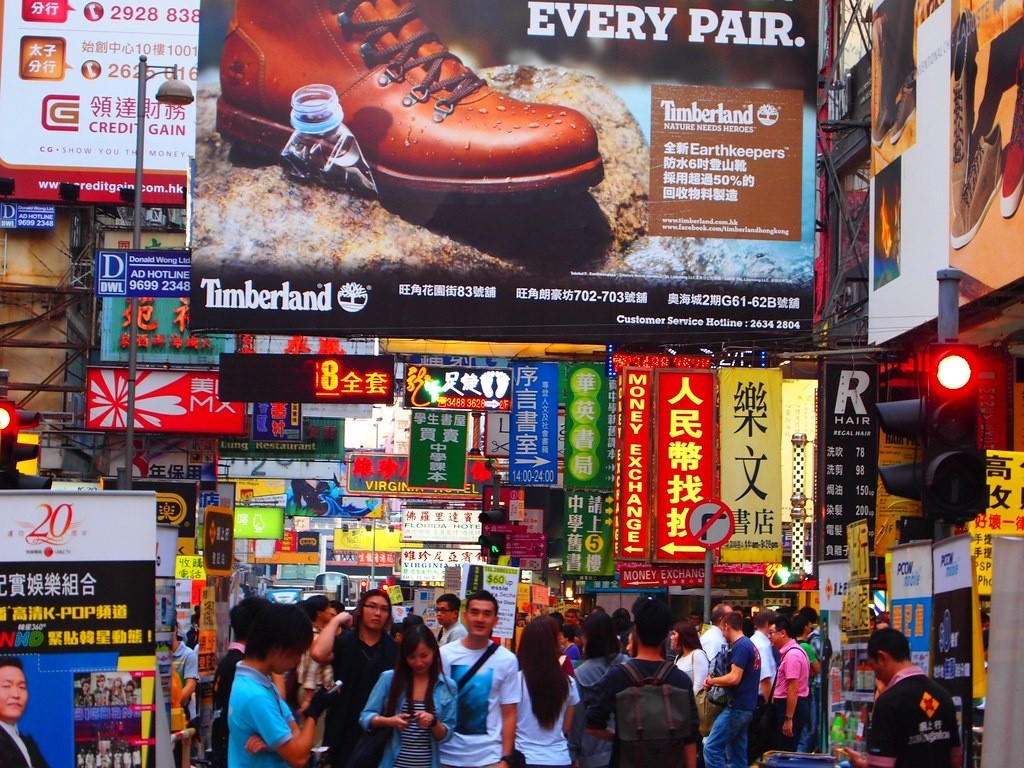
[280,85,393,201]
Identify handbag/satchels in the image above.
[696,688,724,737]
[761,703,774,731]
[348,728,392,768]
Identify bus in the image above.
[314,572,352,605]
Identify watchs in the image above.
[501,756,516,767]
[428,715,437,730]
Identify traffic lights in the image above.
[361,580,367,593]
[874,393,926,518]
[478,532,506,555]
[925,342,990,520]
[776,556,799,583]
[15,409,52,489]
[382,583,389,592]
[0,400,16,490]
[478,509,505,524]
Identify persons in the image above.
[358,607,458,768]
[584,597,703,768]
[309,589,399,768]
[820,617,833,754]
[566,605,637,768]
[434,593,469,646]
[293,596,345,764]
[227,603,341,768]
[0,657,50,768]
[845,627,963,768]
[667,604,820,768]
[211,597,287,768]
[171,604,199,726]
[512,612,581,768]
[75,674,136,707]
[438,590,522,768]
[77,736,142,768]
[973,613,991,710]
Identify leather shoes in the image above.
[217,1,603,193]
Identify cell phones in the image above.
[328,680,343,694]
[836,747,848,755]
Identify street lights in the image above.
[124,55,194,491]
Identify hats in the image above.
[632,597,653,613]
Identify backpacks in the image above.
[808,634,832,681]
[616,662,692,768]
[706,645,731,706]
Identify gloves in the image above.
[303,687,331,722]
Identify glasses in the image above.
[435,607,456,613]
[363,603,390,614]
